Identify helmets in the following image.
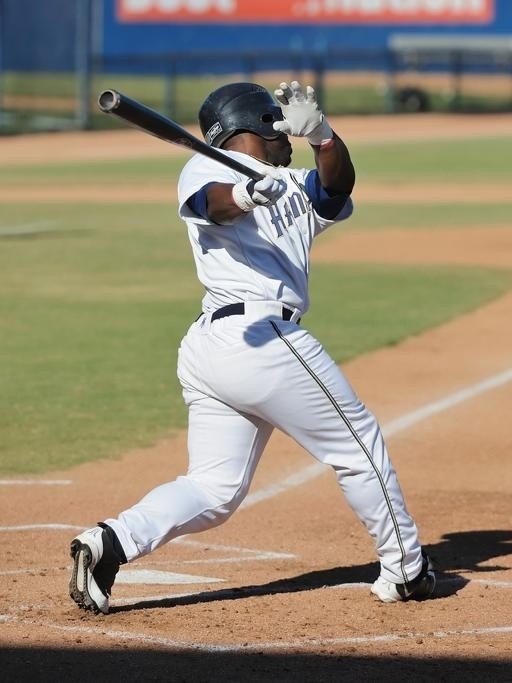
[195,78,289,148]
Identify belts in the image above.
[194,298,303,327]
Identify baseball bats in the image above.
[96,89,265,181]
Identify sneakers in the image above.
[65,520,116,617]
[369,550,467,604]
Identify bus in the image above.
[384,32,512,114]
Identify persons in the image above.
[64,78,445,616]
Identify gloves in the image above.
[268,77,335,151]
[227,166,289,214]
